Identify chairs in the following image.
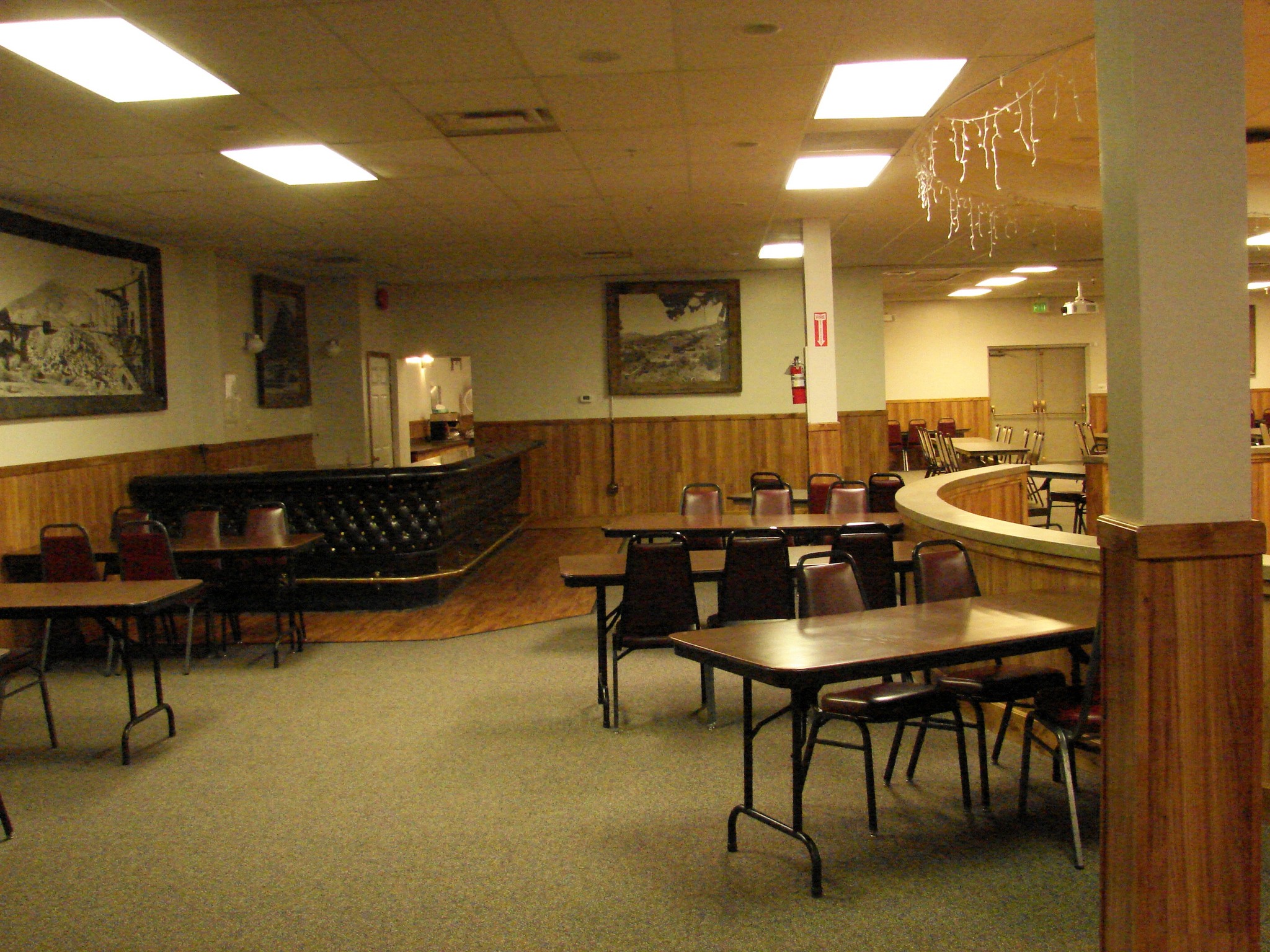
[906,417,1109,478]
[41,506,307,675]
[1250,408,1270,446]
[1019,604,1103,868]
[888,419,905,472]
[614,470,904,733]
[907,539,1076,809]
[795,551,972,834]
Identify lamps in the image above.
[245,332,263,353]
[327,340,340,356]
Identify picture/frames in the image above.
[606,279,743,394]
[0,208,167,420]
[256,275,313,408]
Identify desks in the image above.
[0,577,204,765]
[557,540,912,728]
[5,533,326,666]
[727,488,808,506]
[931,437,1030,466]
[669,586,1106,900]
[1028,462,1085,532]
[602,511,906,539]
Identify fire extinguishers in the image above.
[790,356,807,404]
